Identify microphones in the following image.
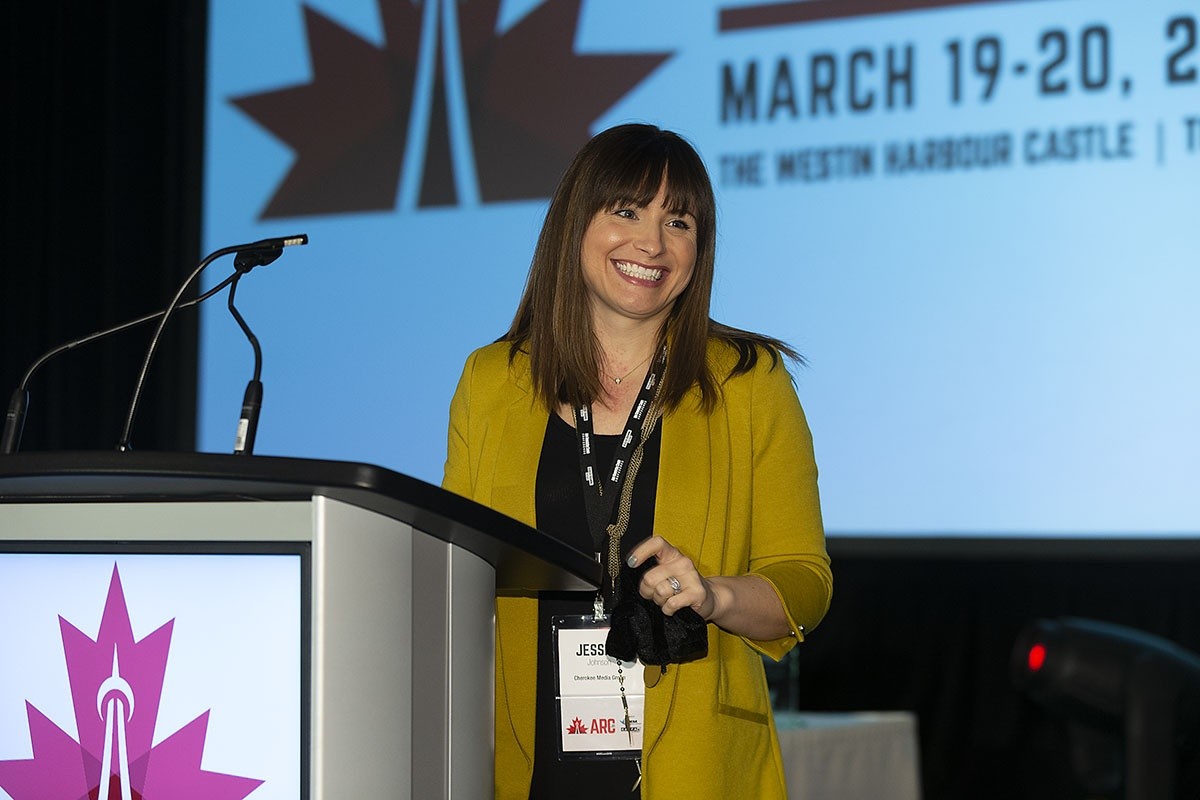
[0,235,309,456]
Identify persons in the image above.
[440,124,834,800]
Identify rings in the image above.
[666,577,681,595]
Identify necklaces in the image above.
[571,316,675,793]
[601,353,654,385]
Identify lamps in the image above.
[1015,617,1200,800]
